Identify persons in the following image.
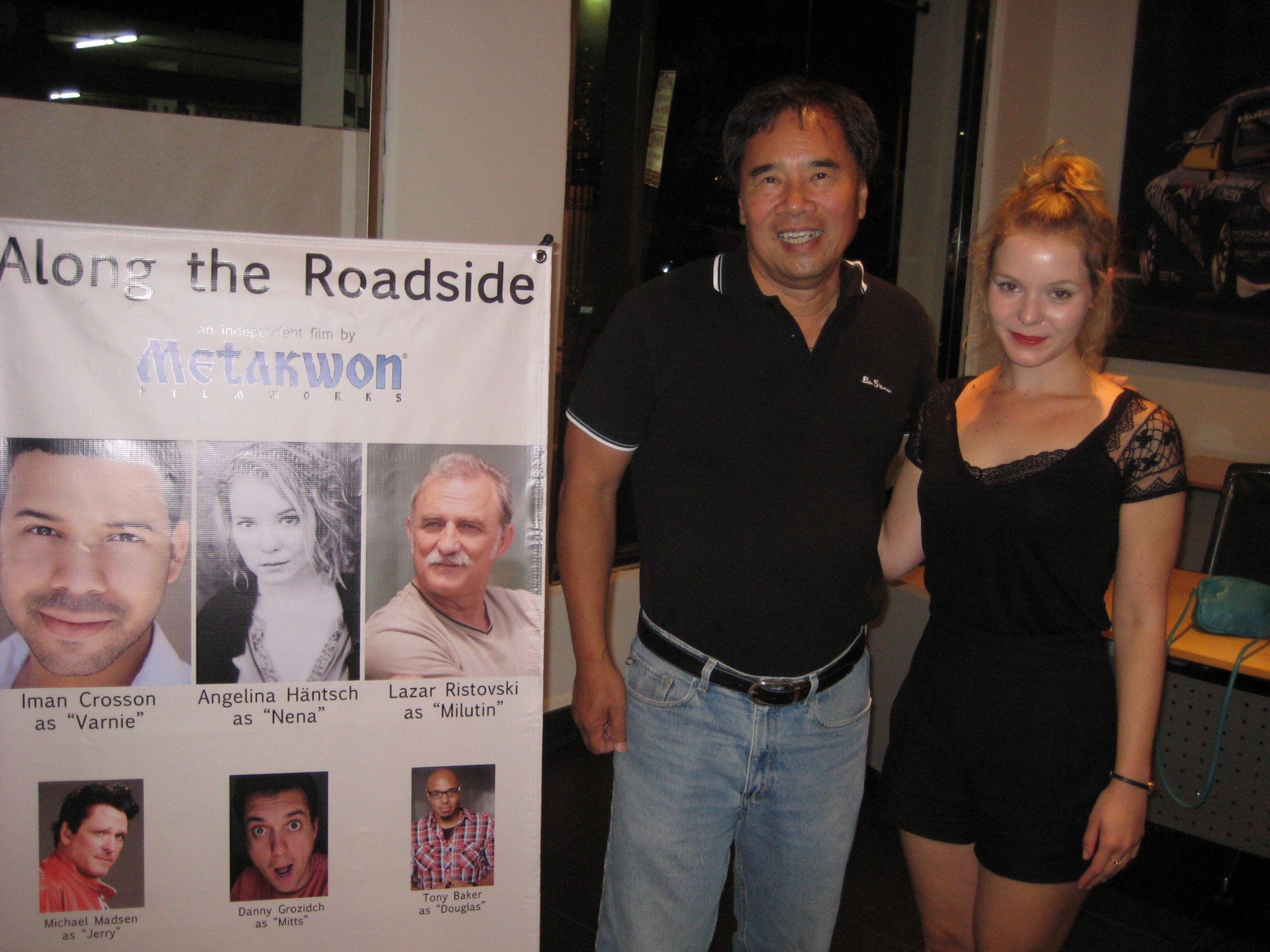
[230,773,328,901]
[557,84,935,951]
[195,441,360,684]
[39,781,140,913]
[364,450,541,681]
[0,438,192,689]
[410,768,494,890]
[875,140,1186,951]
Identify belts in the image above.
[637,603,866,708]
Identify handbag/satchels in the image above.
[1190,573,1270,638]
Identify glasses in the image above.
[426,784,460,800]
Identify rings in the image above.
[1109,858,1122,865]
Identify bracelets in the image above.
[1109,771,1154,793]
[469,882,478,886]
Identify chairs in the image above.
[1202,461,1269,586]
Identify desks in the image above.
[865,565,1270,861]
